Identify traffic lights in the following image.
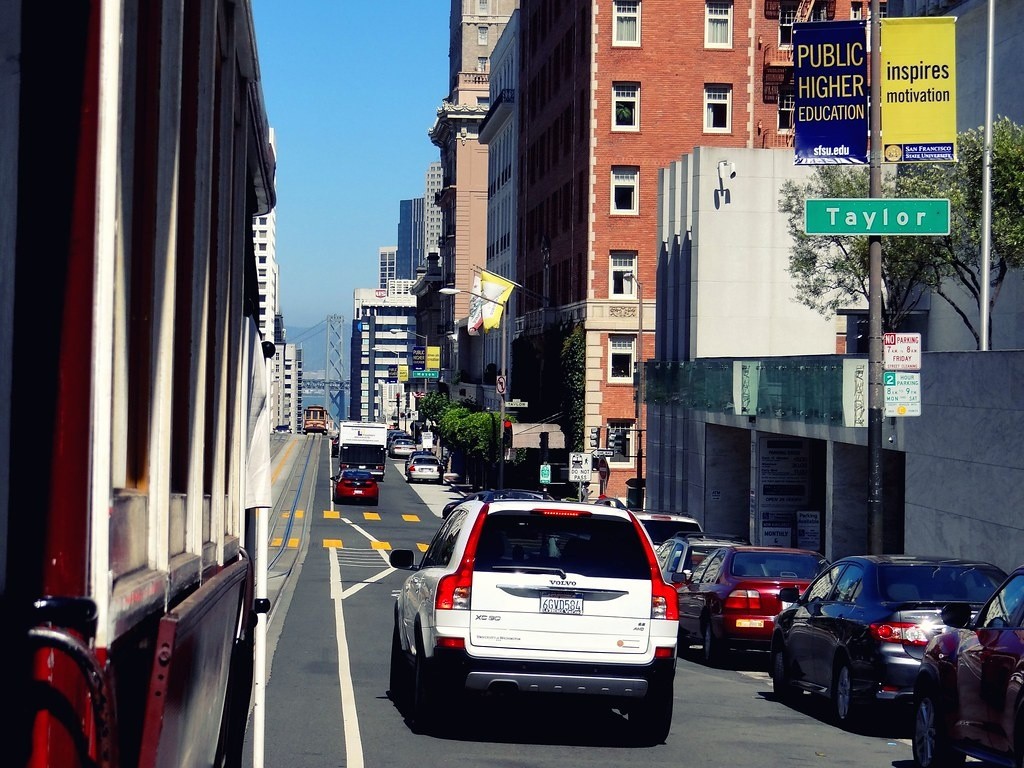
[405,392,412,408]
[614,431,624,455]
[504,420,512,431]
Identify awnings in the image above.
[509,422,566,449]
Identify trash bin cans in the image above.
[626,486,645,511]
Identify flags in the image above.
[467,276,483,335]
[480,270,515,334]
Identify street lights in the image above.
[389,328,428,397]
[438,287,506,491]
[372,347,400,393]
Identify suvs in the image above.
[651,531,753,589]
[627,508,707,553]
[389,491,681,747]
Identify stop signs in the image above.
[599,456,608,481]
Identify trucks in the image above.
[338,420,387,482]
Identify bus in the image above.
[302,404,340,436]
[0,0,279,768]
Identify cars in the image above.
[910,564,1024,768]
[273,425,293,435]
[330,437,340,458]
[769,553,1010,733]
[670,546,831,668]
[388,429,443,485]
[330,470,378,506]
